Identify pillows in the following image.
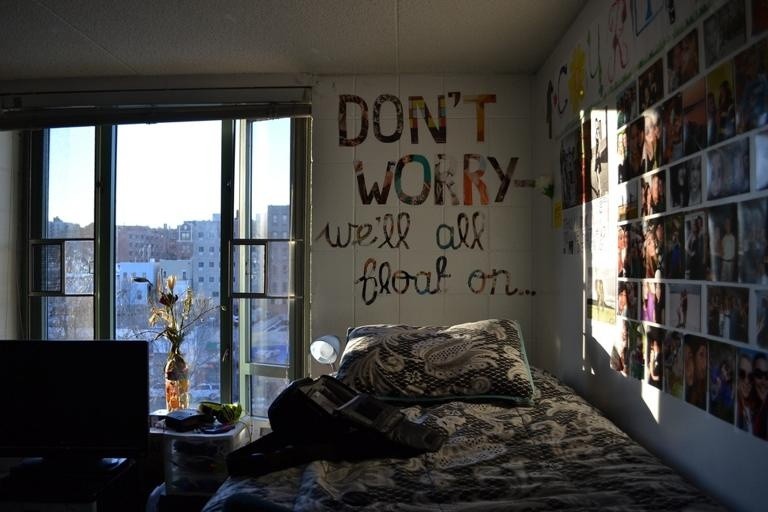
[334,317,537,406]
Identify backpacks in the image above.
[224,376,448,478]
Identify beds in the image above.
[199,367,734,512]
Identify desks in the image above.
[0,455,136,511]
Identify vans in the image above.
[188,382,220,401]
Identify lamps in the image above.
[310,335,342,372]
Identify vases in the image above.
[163,353,188,412]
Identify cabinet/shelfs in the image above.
[147,415,247,498]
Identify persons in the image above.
[561,37,768,442]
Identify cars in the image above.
[149,383,166,397]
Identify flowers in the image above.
[135,275,226,380]
